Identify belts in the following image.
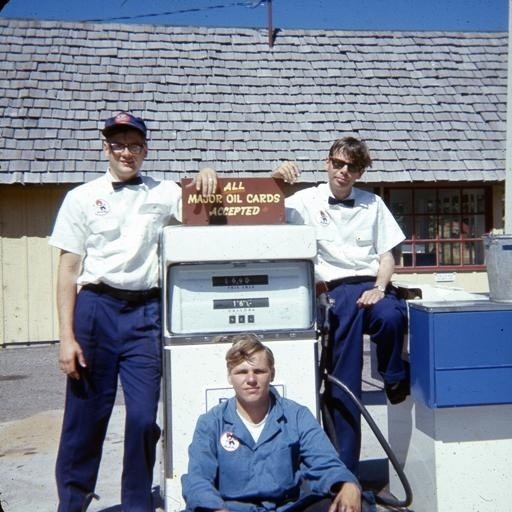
[83,282,163,303]
[324,276,380,290]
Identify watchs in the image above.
[373,284,386,293]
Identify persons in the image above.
[47,113,218,511]
[181,335,378,512]
[272,136,410,479]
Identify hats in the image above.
[101,112,147,137]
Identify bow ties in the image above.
[111,177,143,191]
[328,196,355,208]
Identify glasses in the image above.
[329,156,363,172]
[108,141,144,154]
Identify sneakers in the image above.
[382,359,410,404]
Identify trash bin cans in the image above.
[481,232,512,304]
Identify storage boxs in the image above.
[406,294,512,410]
